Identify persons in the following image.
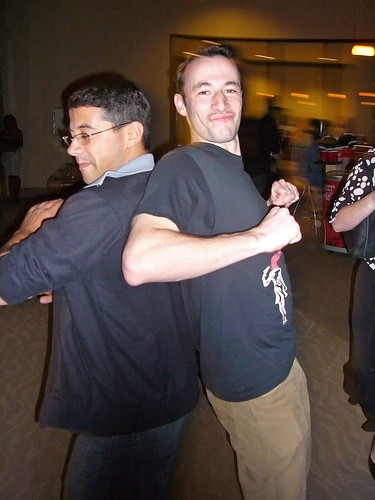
[120,43,310,500]
[0,113,24,203]
[259,106,281,194]
[328,149,375,478]
[0,69,196,500]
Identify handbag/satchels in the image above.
[342,158,375,259]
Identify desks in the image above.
[48,164,83,199]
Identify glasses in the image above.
[62,120,134,147]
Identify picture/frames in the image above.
[52,106,64,135]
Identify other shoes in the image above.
[362,419,375,431]
